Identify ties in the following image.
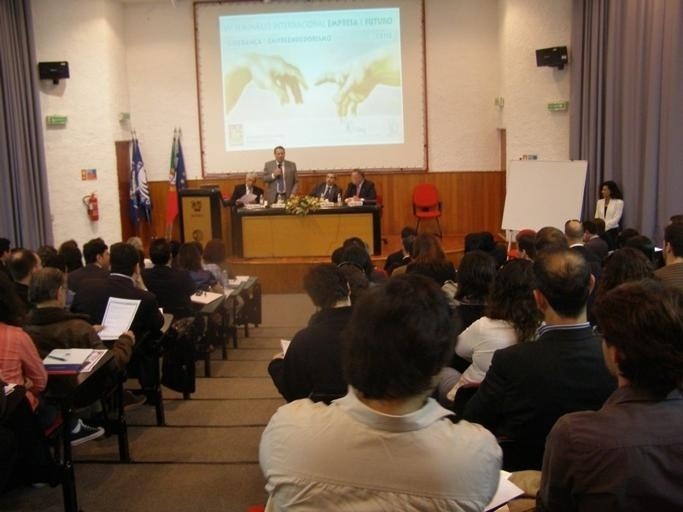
[277,164,284,192]
[356,185,360,197]
[324,187,329,199]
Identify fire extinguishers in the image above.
[83,190,98,221]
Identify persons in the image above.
[459,248,617,470]
[515,228,536,258]
[7,250,43,302]
[24,267,147,412]
[70,237,110,289]
[582,222,607,254]
[0,237,11,275]
[201,239,225,282]
[564,218,588,256]
[127,236,154,269]
[594,180,625,232]
[70,243,164,347]
[258,273,502,512]
[268,264,354,403]
[262,147,299,204]
[172,244,216,291]
[0,270,105,490]
[407,233,455,284]
[230,171,264,206]
[652,223,683,290]
[437,258,546,411]
[589,247,660,296]
[536,280,683,512]
[38,240,83,272]
[143,244,195,317]
[331,226,416,280]
[309,172,342,203]
[667,214,683,225]
[534,226,569,253]
[343,169,376,205]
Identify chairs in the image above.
[453,382,483,417]
[69,363,133,467]
[412,182,443,239]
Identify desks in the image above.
[176,186,223,250]
[219,287,236,362]
[235,199,381,260]
[37,347,118,512]
[228,280,247,350]
[236,274,261,329]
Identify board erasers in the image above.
[523,155,538,161]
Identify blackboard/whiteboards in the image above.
[500,159,591,239]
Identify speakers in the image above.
[536,46,569,67]
[39,61,70,80]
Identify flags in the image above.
[129,137,153,228]
[170,128,187,186]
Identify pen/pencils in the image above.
[47,354,66,362]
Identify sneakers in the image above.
[70,417,104,446]
[121,389,146,412]
